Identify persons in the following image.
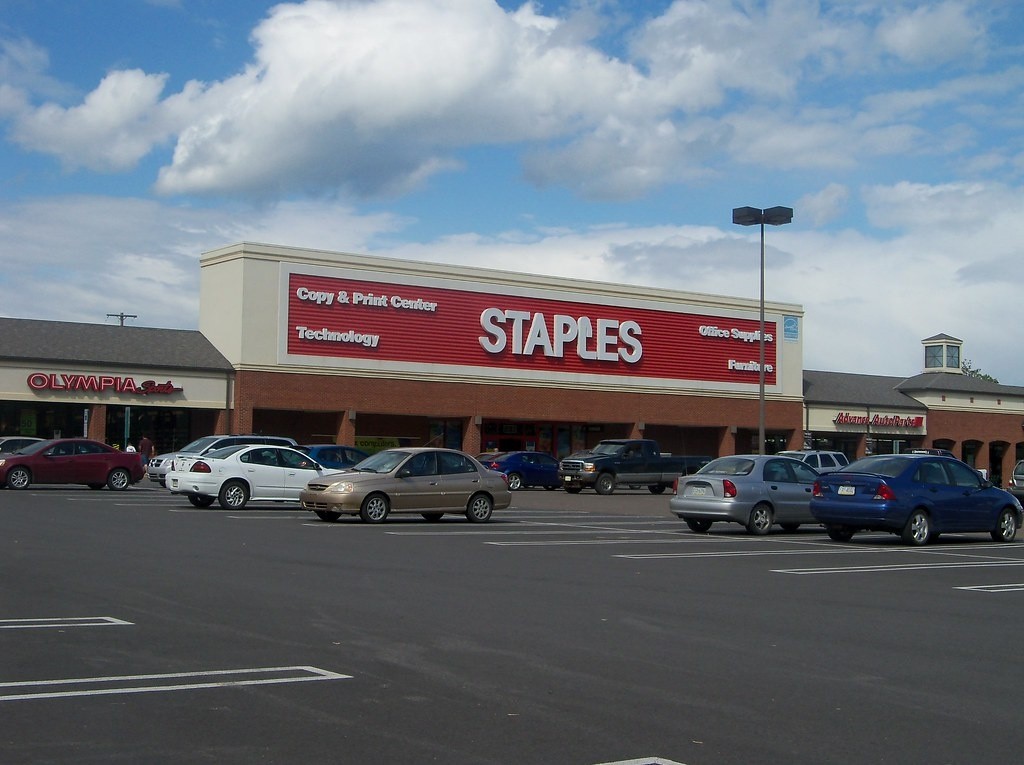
[139,433,154,477]
[127,441,136,452]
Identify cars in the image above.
[165,444,346,510]
[903,448,957,459]
[0,437,49,460]
[479,451,563,491]
[1007,460,1024,495]
[810,454,1024,548]
[0,439,146,491]
[289,444,371,469]
[299,447,511,524]
[670,455,821,536]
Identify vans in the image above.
[148,436,298,487]
[774,450,850,476]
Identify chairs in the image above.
[768,471,781,481]
[923,464,938,483]
[248,454,264,463]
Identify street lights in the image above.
[733,206,793,455]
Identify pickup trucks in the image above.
[559,439,713,495]
